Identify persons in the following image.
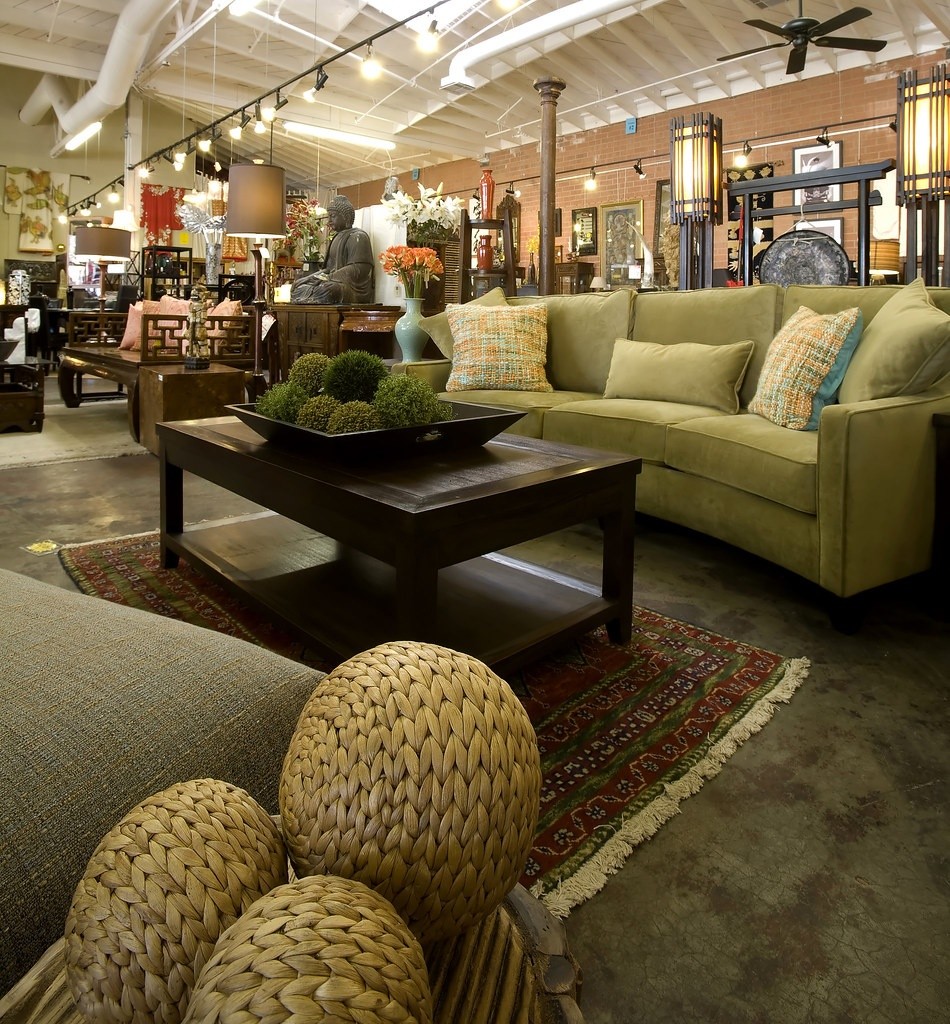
[288,196,374,303]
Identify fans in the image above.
[716,0,887,74]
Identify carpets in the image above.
[58,510,805,916]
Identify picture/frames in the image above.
[498,139,861,291]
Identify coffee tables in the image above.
[154,410,642,688]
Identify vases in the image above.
[7,269,31,306]
[395,299,429,362]
[297,260,329,281]
[478,235,494,273]
[480,169,495,220]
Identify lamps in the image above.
[52,0,511,223]
[670,112,722,291]
[225,164,286,402]
[75,227,132,339]
[895,67,949,288]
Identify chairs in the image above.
[0,304,60,437]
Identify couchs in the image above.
[0,566,328,995]
[394,272,949,593]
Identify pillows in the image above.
[839,277,950,405]
[419,287,552,393]
[603,335,755,415]
[747,305,865,430]
[119,293,244,356]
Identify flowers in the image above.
[377,245,444,298]
[382,183,465,247]
[286,197,330,261]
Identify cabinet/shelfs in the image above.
[269,302,383,382]
[0,250,302,370]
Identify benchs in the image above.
[57,304,257,443]
